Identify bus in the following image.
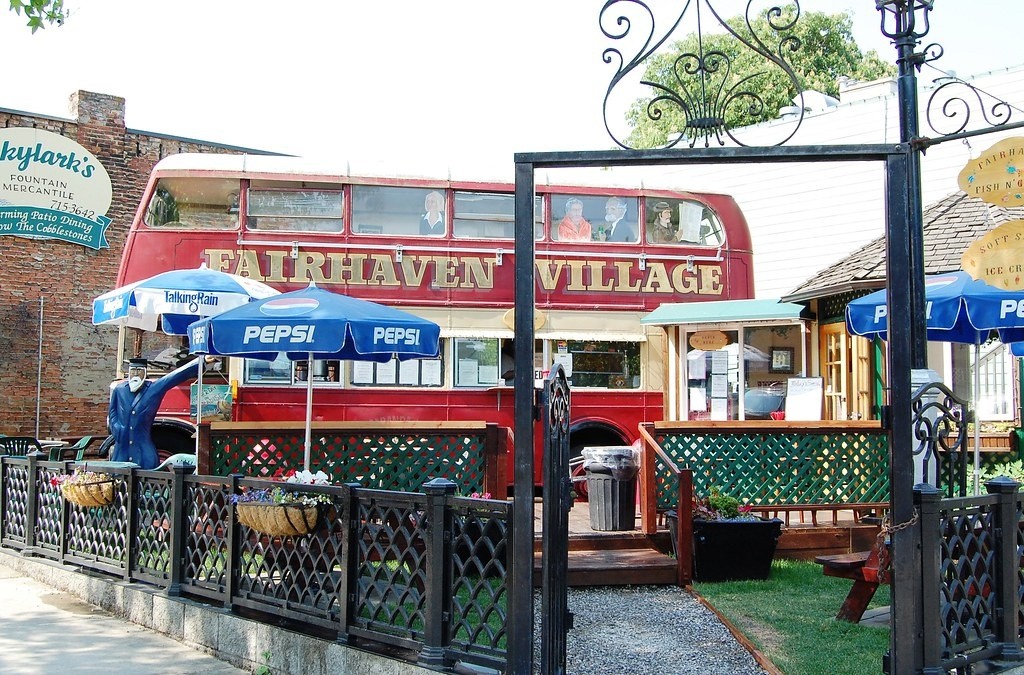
[99,151,758,499]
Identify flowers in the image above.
[224,465,336,508]
[675,483,756,519]
[49,463,120,488]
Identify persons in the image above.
[225,202,239,229]
[109,353,215,469]
[501,339,514,386]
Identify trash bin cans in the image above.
[581,445,640,532]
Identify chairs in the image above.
[744,387,785,420]
[0,433,200,554]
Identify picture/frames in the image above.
[768,346,795,375]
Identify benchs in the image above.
[814,521,1024,628]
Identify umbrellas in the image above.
[92,262,283,475]
[187,280,440,470]
[845,271,1024,528]
[723,342,771,362]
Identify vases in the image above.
[663,510,785,584]
